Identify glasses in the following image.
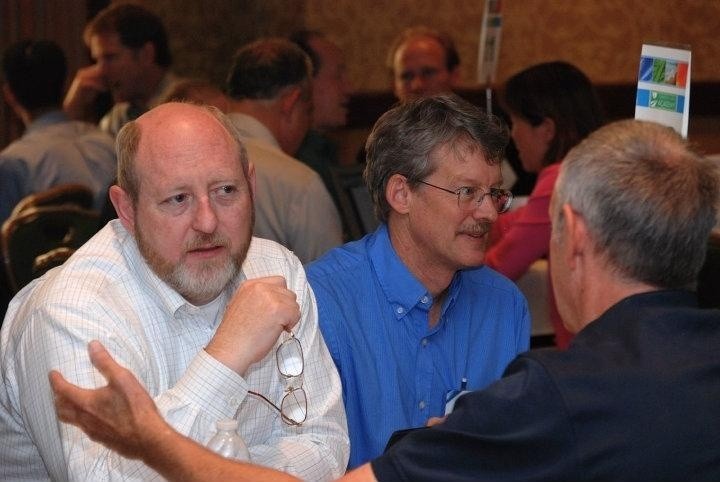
[248,330,308,427]
[419,181,513,213]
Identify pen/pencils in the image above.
[462,378,468,390]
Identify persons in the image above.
[302,90,531,472]
[64,1,194,137]
[0,40,118,225]
[221,31,379,266]
[388,26,460,103]
[0,102,350,481]
[49,119,720,482]
[482,61,607,351]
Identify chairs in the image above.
[0,205,103,298]
